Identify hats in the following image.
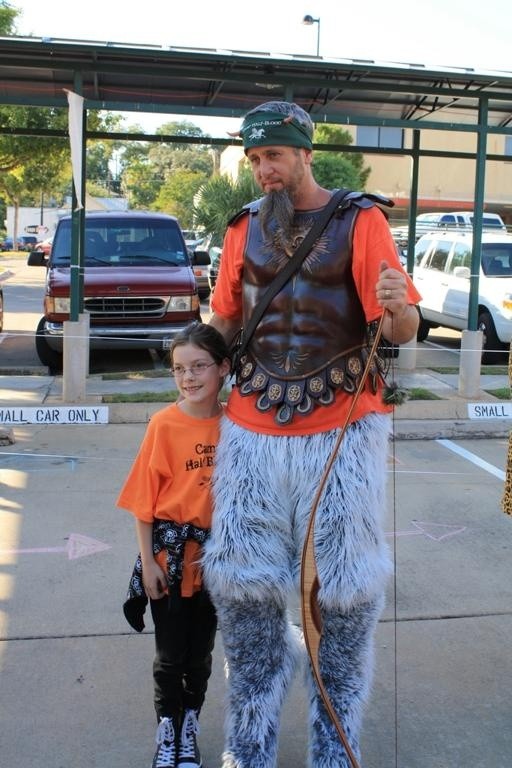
[241,100,315,158]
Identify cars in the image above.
[388,210,512,365]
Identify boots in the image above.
[178,706,203,767]
[151,712,176,767]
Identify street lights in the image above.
[303,14,320,128]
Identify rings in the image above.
[381,286,394,300]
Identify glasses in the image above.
[169,361,217,378]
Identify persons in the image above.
[204,98,421,767]
[113,320,235,766]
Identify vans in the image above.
[25,208,214,369]
